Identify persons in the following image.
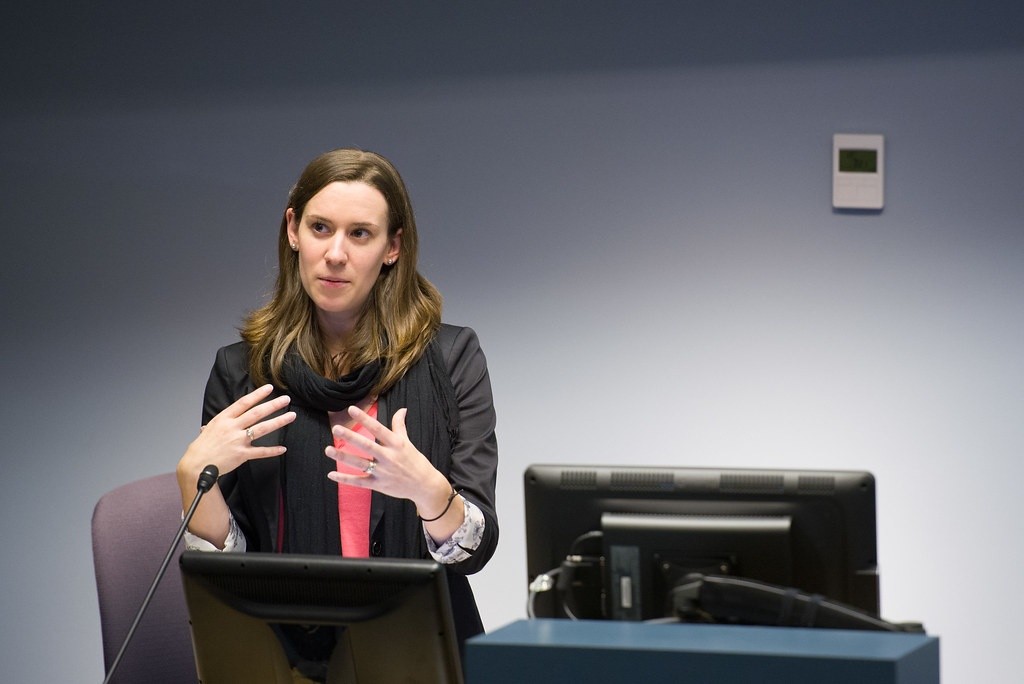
[178,151,520,648]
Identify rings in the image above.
[363,459,377,474]
[246,427,255,441]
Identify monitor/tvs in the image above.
[523,463,881,633]
[177,550,466,684]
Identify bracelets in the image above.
[416,482,463,522]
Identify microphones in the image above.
[102,464,220,684]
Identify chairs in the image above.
[90,471,195,684]
